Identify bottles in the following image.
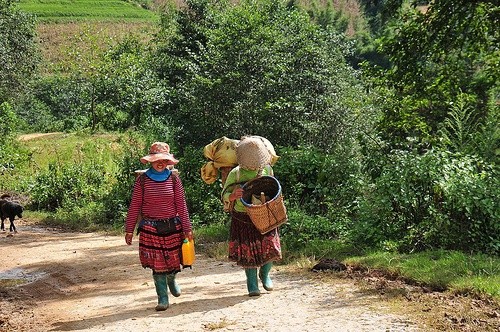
[260,192,265,203]
[181,238,195,265]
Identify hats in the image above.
[140,142,179,165]
[237,136,276,171]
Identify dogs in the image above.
[0,194,24,233]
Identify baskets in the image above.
[240,175,288,235]
[134,169,178,222]
[223,167,235,215]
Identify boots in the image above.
[167,273,181,297]
[155,274,170,311]
[259,261,274,291]
[245,267,261,296]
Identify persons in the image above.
[221,137,282,296]
[124,142,194,311]
